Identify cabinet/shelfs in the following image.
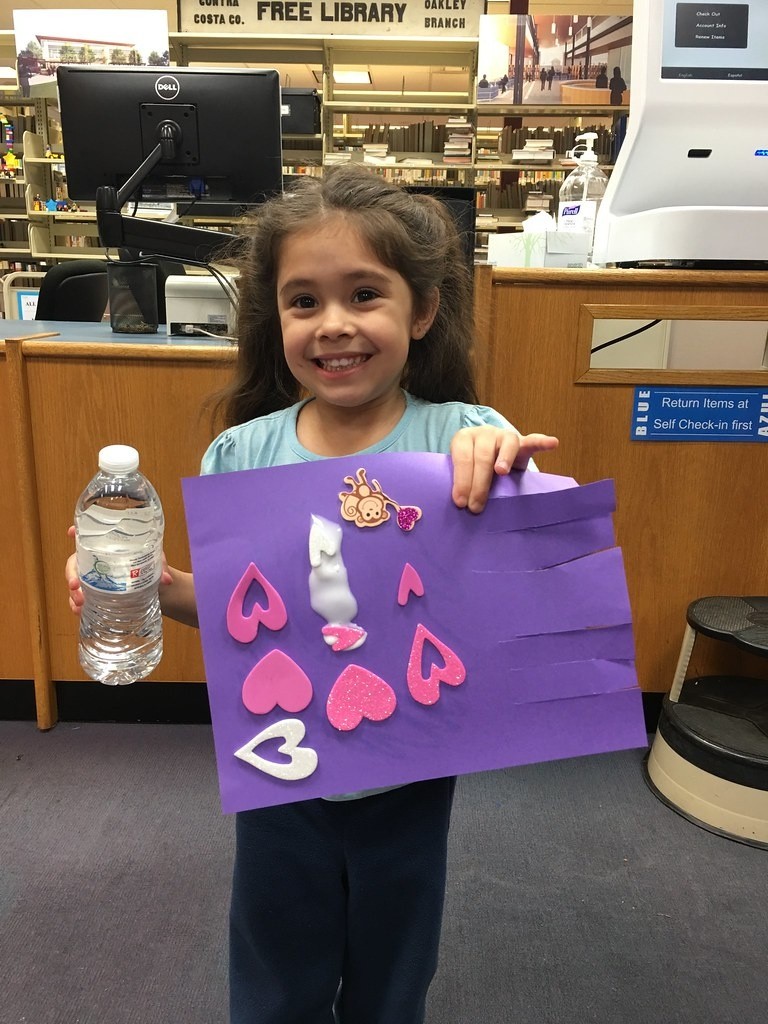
[0,33,767,732]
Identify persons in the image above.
[65,165,561,1024]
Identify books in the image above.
[323,117,475,166]
[283,166,574,227]
[474,232,497,262]
[479,124,614,166]
[0,111,244,287]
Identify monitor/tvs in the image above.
[55,65,282,267]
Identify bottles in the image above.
[78,444,164,687]
[557,134,618,270]
